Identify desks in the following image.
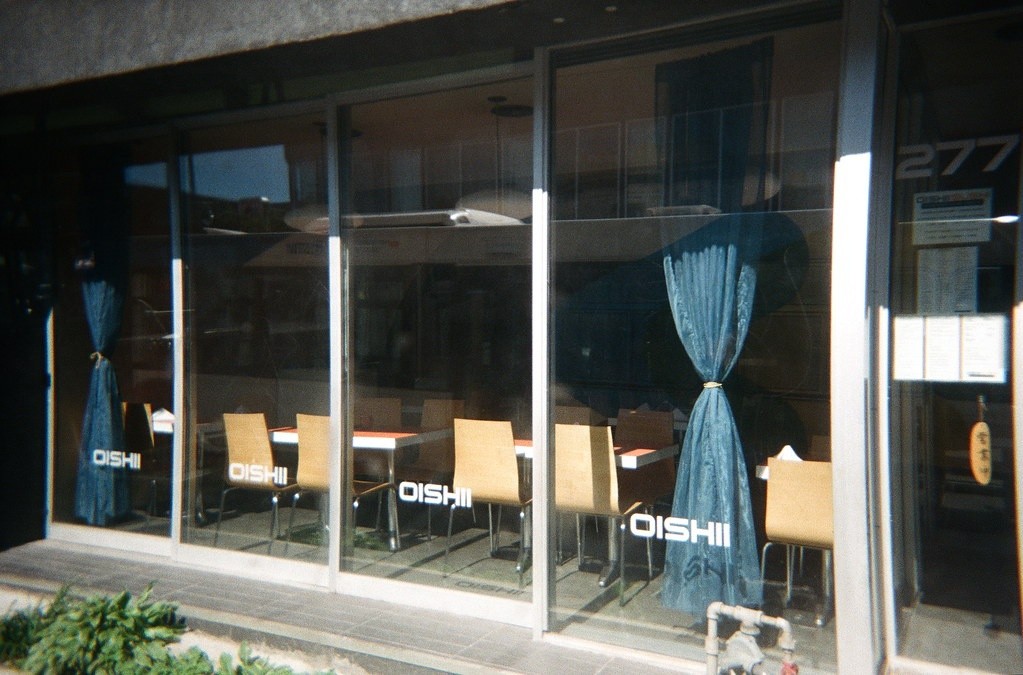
[513,429,681,588]
[193,414,225,528]
[756,449,847,628]
[267,423,454,551]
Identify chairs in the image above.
[118,394,835,628]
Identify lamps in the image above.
[281,119,364,234]
[453,95,531,221]
